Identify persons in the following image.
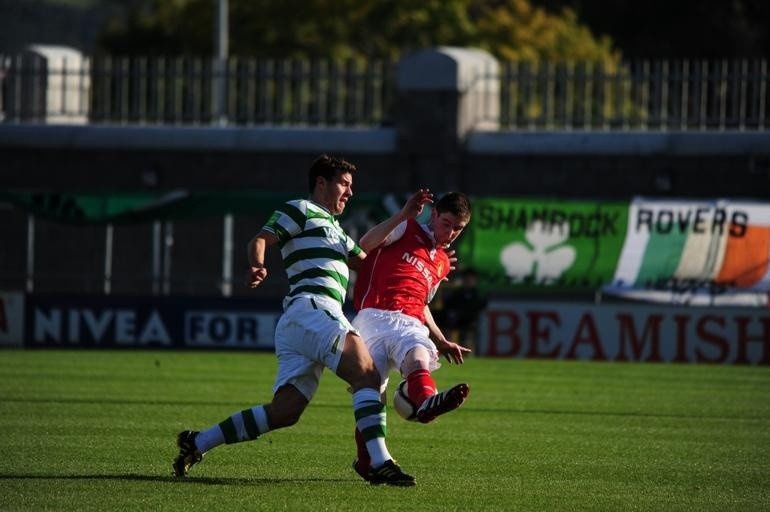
[352,187,474,488]
[173,155,418,489]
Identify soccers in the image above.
[394,378,438,421]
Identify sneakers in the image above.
[353,458,417,487]
[417,383,472,424]
[173,428,201,477]
